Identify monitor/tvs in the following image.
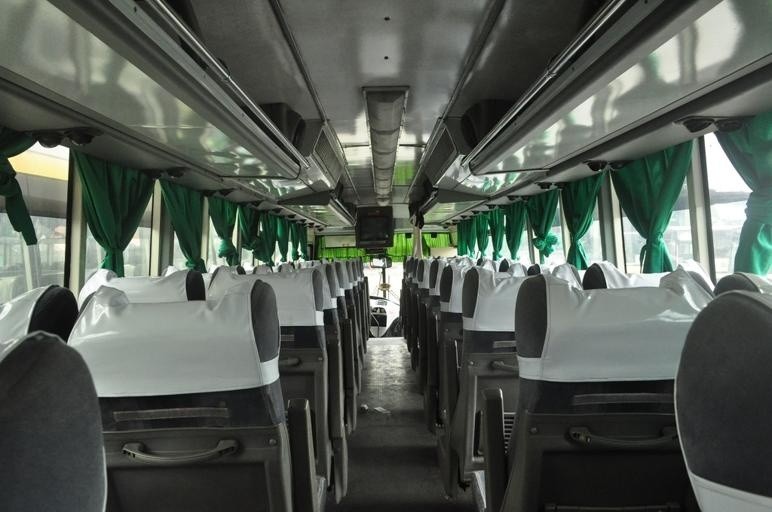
[354,215,394,247]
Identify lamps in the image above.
[361,86,410,195]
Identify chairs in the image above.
[1,256,370,511]
[399,253,771,511]
